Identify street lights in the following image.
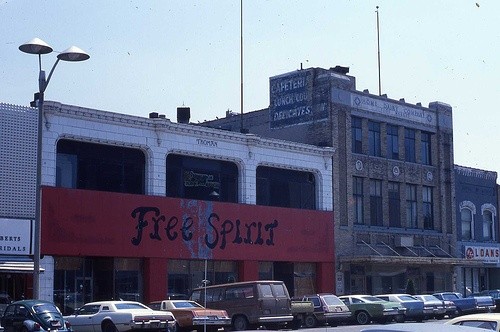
[17,37,92,300]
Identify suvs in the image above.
[186,279,295,332]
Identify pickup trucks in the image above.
[430,291,495,317]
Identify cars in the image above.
[289,286,500,332]
[337,294,407,326]
[61,300,176,332]
[0,298,73,332]
[413,293,458,321]
[144,299,232,332]
[374,294,424,323]
